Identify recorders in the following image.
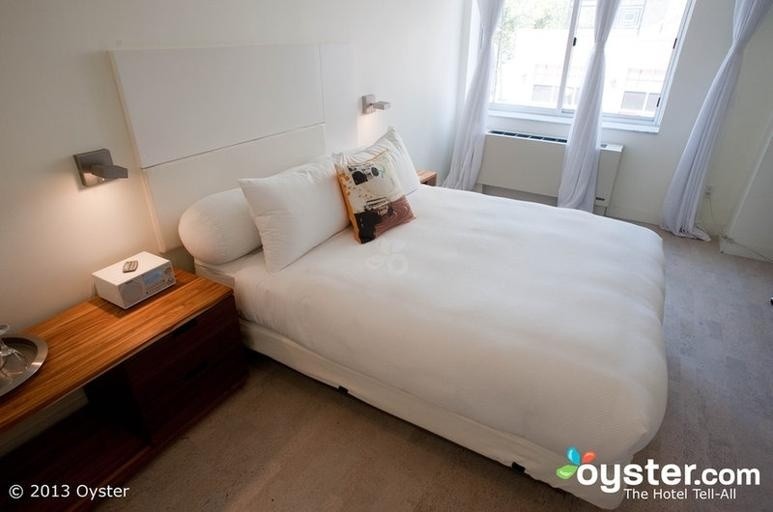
[91,251,177,310]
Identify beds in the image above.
[91,30,674,511]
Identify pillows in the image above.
[331,149,418,246]
[173,163,307,267]
[231,136,356,279]
[329,122,422,197]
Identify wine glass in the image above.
[0,322,29,383]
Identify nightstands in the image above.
[412,166,441,188]
[0,261,254,511]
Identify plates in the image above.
[0,334,49,400]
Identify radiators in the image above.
[467,129,626,218]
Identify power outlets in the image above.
[702,183,714,199]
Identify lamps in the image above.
[70,146,130,191]
[361,93,392,116]
